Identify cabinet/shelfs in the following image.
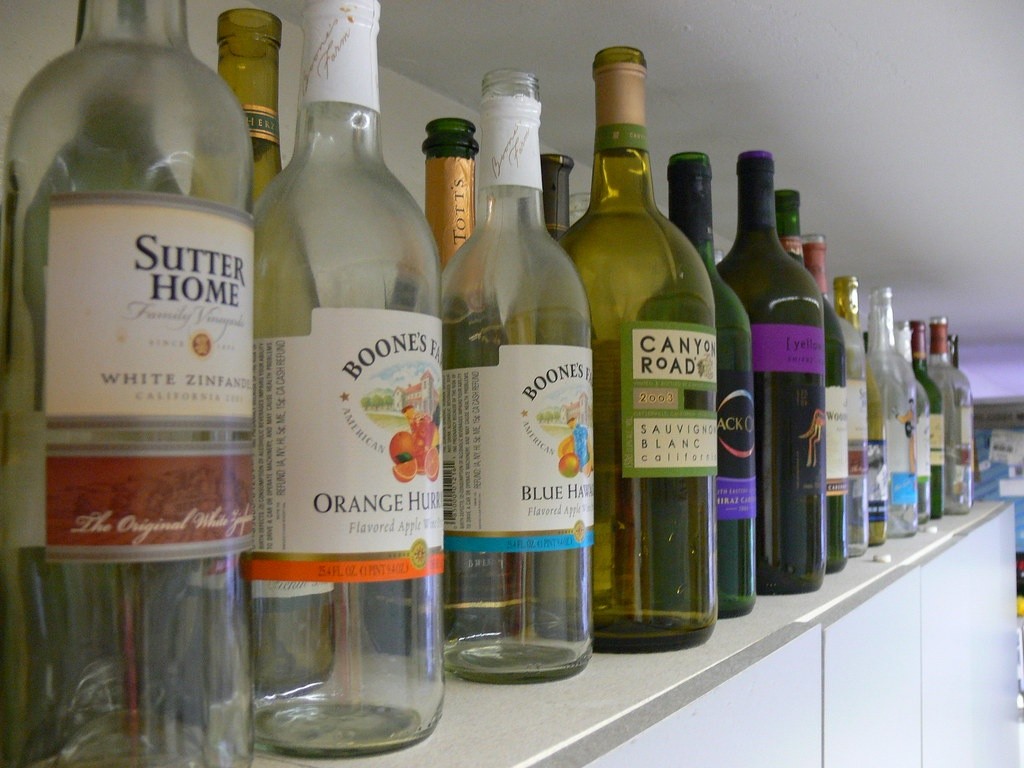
[527,500,1024,768]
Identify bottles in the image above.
[0,0,254,768]
[717,148,826,596]
[207,0,438,757]
[559,46,718,655]
[661,148,761,616]
[420,67,592,689]
[776,187,976,574]
